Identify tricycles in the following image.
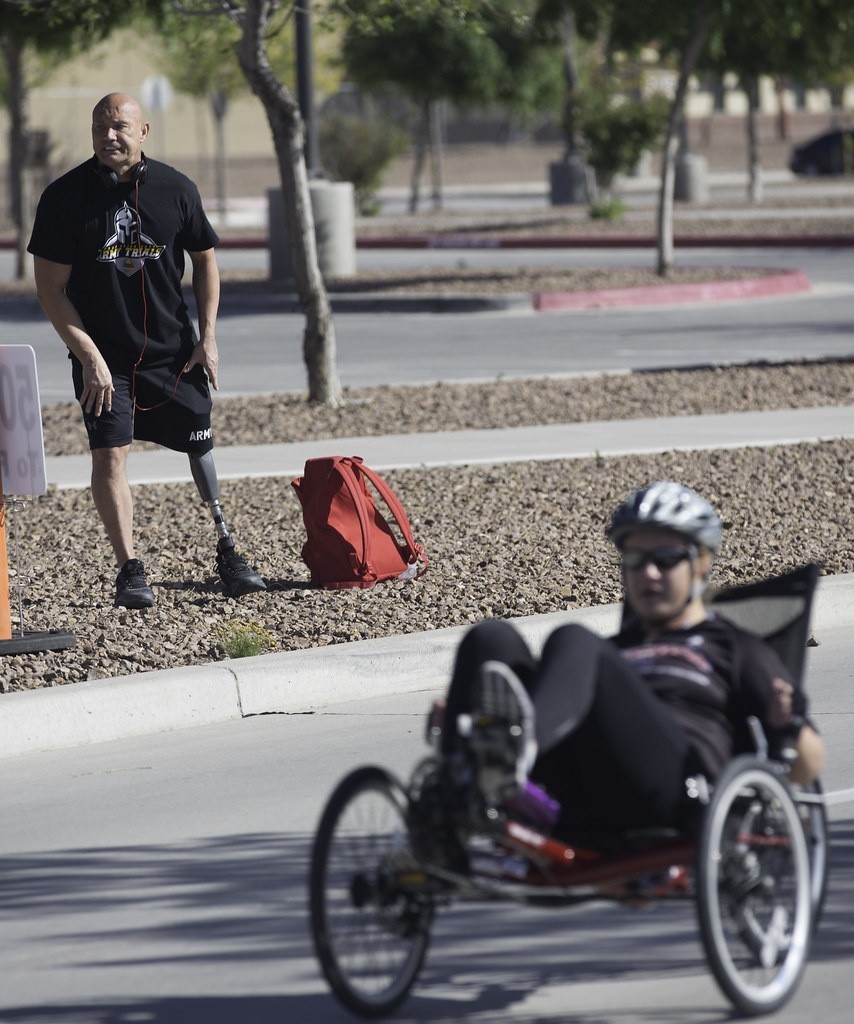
[308,562,832,1021]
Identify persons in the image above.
[25,91,268,609]
[426,482,824,864]
